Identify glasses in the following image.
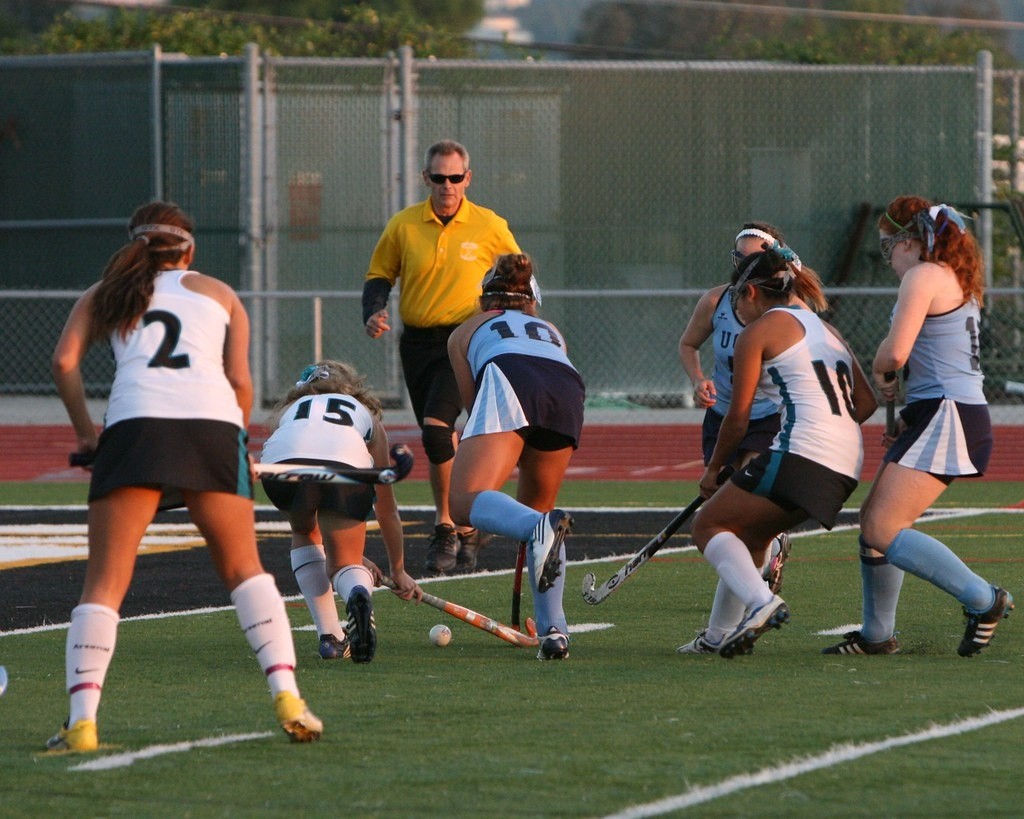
[879,231,921,261]
[728,278,767,311]
[426,168,467,185]
[729,248,746,268]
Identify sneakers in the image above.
[820,630,903,655]
[527,509,575,594]
[673,614,737,655]
[454,528,489,571]
[424,522,461,574]
[719,594,791,659]
[346,584,379,665]
[955,585,1015,658]
[28,715,100,760]
[273,691,325,743]
[319,627,352,661]
[761,532,792,594]
[535,625,571,661]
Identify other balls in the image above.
[429,623,453,647]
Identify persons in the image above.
[448,254,585,658]
[261,358,424,663]
[678,221,879,661]
[30,198,323,756]
[819,196,1017,657]
[361,139,522,574]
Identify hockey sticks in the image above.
[883,370,898,438]
[580,464,737,606]
[66,442,415,487]
[368,568,540,648]
